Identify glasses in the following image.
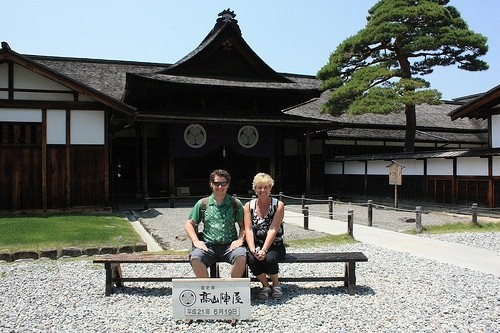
[212,180,229,187]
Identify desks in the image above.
[176,187,190,196]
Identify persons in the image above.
[244,173,284,300]
[184,170,247,278]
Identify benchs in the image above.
[93,252,368,296]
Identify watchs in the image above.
[264,249,268,253]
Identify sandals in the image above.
[259,285,272,300]
[272,286,284,299]
